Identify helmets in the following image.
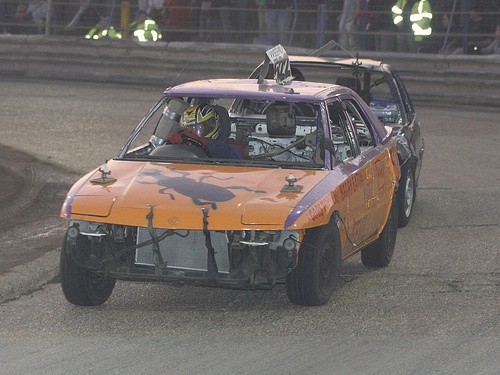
[179,103,220,140]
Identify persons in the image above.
[435,15,460,54]
[161,102,244,159]
[466,5,499,54]
[12,2,370,51]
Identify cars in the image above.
[248,41,424,227]
[60,43,402,308]
